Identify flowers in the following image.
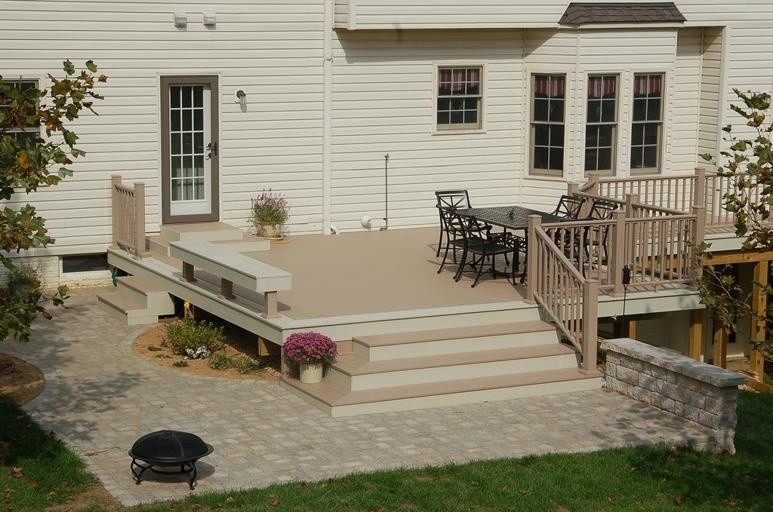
[245,187,290,236]
[280,330,340,369]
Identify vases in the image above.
[298,361,324,383]
[253,220,284,241]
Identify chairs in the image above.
[434,188,618,289]
